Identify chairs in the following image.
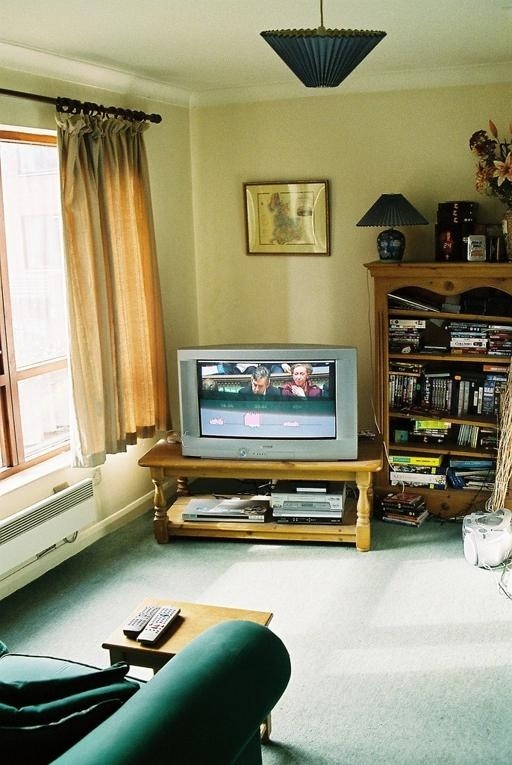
[0,618,291,765]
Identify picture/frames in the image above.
[243,180,332,257]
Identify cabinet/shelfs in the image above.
[138,441,385,553]
[362,262,512,523]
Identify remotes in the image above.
[122,604,161,636]
[136,605,182,646]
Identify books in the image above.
[386,294,438,312]
[380,491,429,529]
[389,315,511,494]
[402,301,428,311]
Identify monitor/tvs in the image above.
[176,342,359,462]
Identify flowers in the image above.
[468,120,512,208]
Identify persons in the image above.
[220,360,329,375]
[275,363,321,400]
[238,365,282,396]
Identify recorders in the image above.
[461,507,512,568]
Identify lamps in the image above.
[260,1,387,89]
[355,193,430,264]
